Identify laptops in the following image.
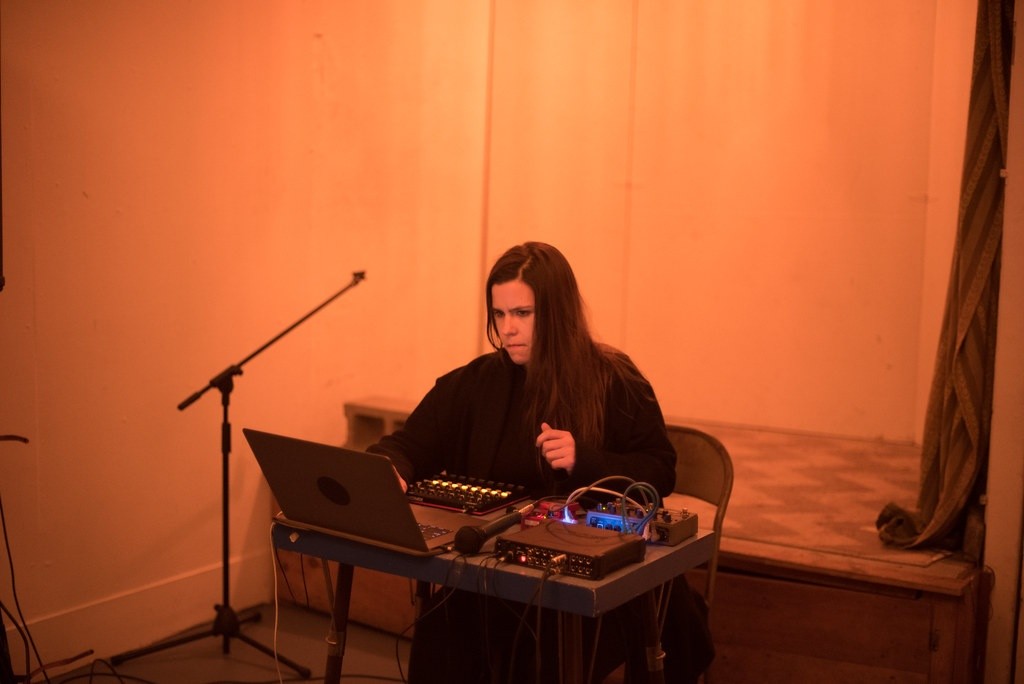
[243,428,491,554]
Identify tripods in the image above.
[109,271,365,679]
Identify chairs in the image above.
[666,423,735,684]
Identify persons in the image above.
[364,240,716,683]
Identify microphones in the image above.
[454,501,538,555]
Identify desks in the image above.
[272,498,715,684]
